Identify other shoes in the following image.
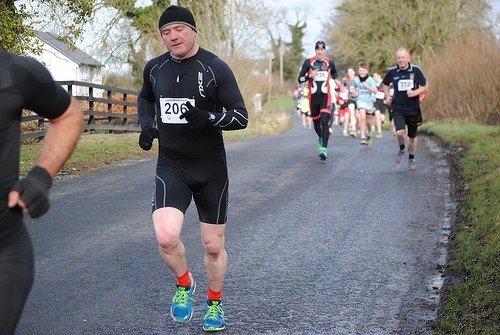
[342,129,348,137]
[350,131,357,138]
[376,133,382,138]
[329,128,333,135]
[360,137,366,144]
[366,136,371,144]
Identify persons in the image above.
[383,47,427,170]
[329,61,396,146]
[298,41,341,161]
[294,86,312,130]
[0,50,85,335]
[137,6,248,331]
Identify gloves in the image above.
[180,101,208,127]
[139,126,159,151]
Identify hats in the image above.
[158,5,197,33]
[315,41,326,49]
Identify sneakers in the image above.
[317,137,323,149]
[397,151,405,164]
[202,299,227,331]
[319,148,327,160]
[169,272,197,325]
[408,154,417,169]
